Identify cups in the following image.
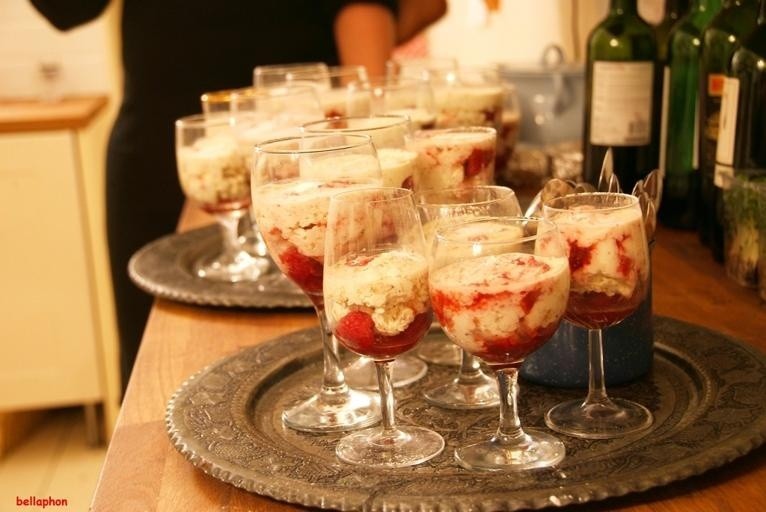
[720,169,766,289]
[749,180,766,303]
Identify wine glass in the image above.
[495,86,521,171]
[299,116,428,391]
[533,192,653,441]
[428,217,571,473]
[413,127,497,367]
[323,186,446,470]
[385,56,461,89]
[434,81,507,184]
[174,110,269,282]
[250,132,384,434]
[253,63,331,86]
[285,64,372,129]
[415,186,526,412]
[346,75,439,190]
[200,82,326,141]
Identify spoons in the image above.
[540,144,662,246]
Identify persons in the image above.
[31,0,396,408]
[396,0,447,50]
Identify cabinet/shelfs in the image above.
[427,0,665,77]
[2,96,122,450]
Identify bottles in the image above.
[654,0,692,226]
[582,0,663,245]
[659,0,724,230]
[700,0,758,248]
[705,1,766,264]
